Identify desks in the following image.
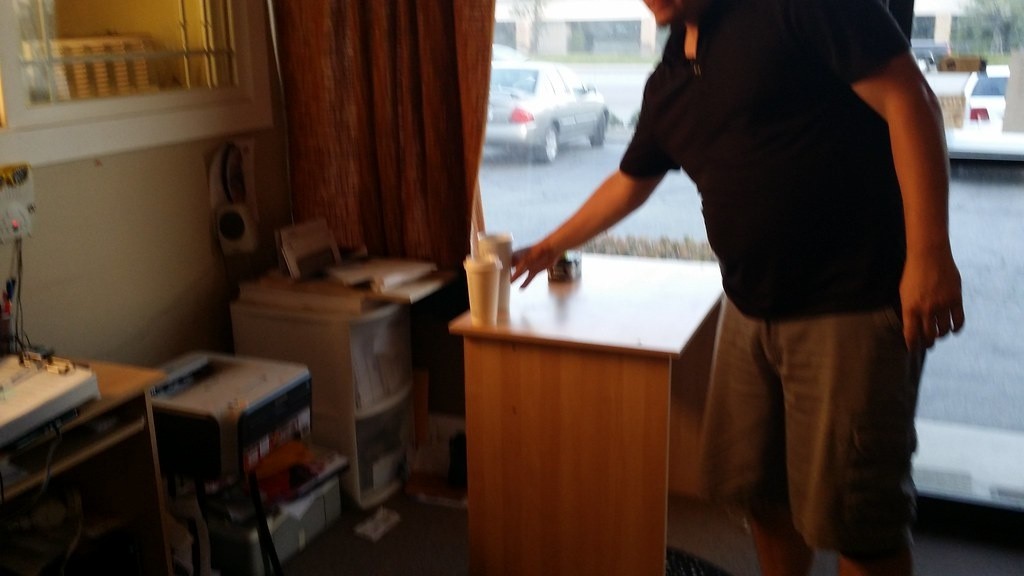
[1,363,169,576]
[450,253,725,576]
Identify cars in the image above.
[477,61,608,156]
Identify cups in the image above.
[481,234,513,313]
[464,257,503,326]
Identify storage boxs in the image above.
[207,475,340,576]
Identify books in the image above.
[332,260,437,294]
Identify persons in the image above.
[509,0,966,576]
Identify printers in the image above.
[145,348,314,490]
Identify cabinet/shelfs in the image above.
[230,282,418,506]
